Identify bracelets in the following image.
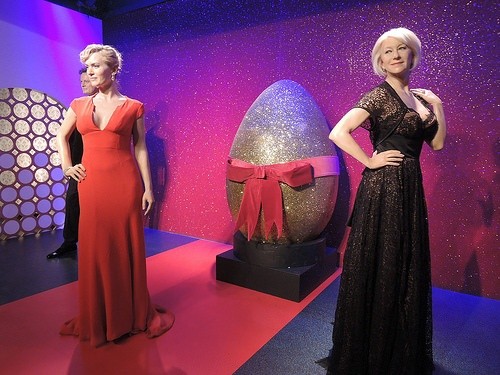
[63,167,71,180]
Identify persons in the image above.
[55,44,156,335]
[46,67,99,259]
[329,27,446,364]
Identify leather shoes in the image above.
[46,244,78,259]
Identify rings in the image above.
[421,89,425,94]
